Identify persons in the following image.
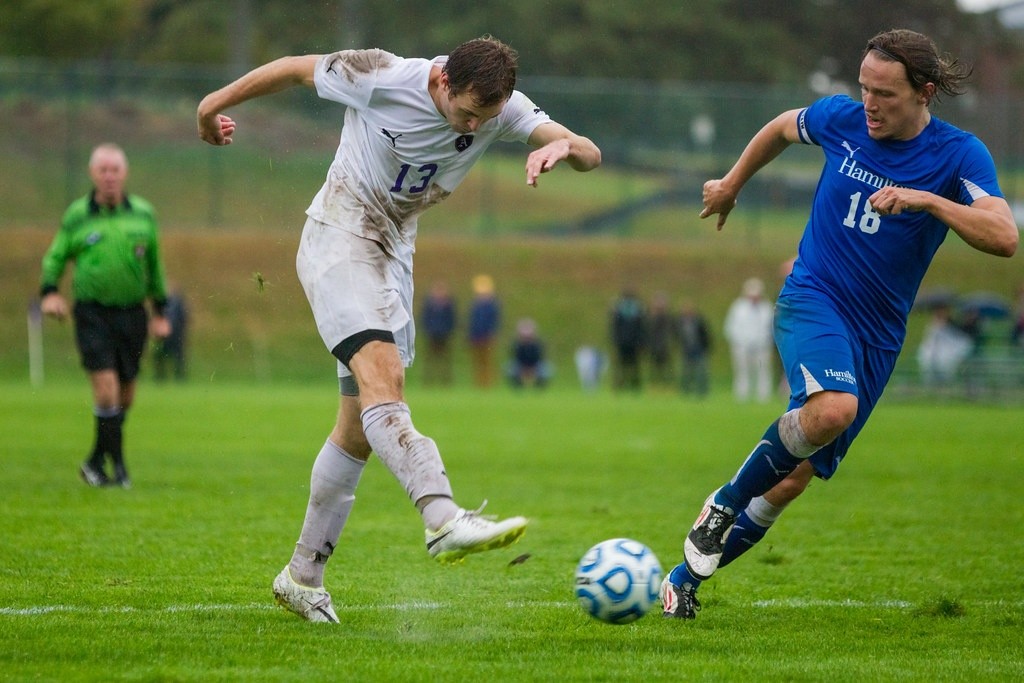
[194,41,603,637]
[15,262,1020,417]
[658,29,1018,631]
[37,141,171,490]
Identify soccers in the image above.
[576,539,663,627]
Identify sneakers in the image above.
[78,460,108,485]
[659,567,695,622]
[274,566,340,626]
[427,514,529,566]
[683,485,738,581]
